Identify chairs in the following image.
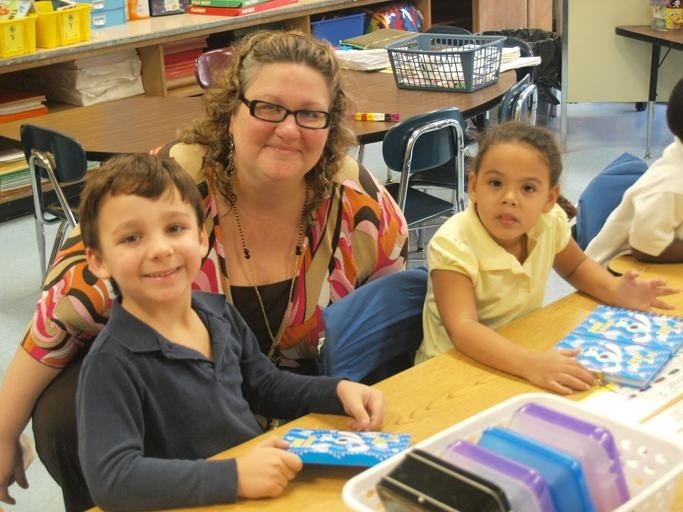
[18,123,92,291]
[193,50,250,88]
[383,109,467,254]
[408,73,538,252]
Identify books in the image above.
[0,89,54,194]
[332,27,541,87]
[280,429,410,467]
[552,303,681,390]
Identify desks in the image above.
[81,253,683,512]
[614,25,683,162]
[0,57,518,162]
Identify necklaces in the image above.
[224,164,310,361]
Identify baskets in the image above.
[385,33,507,93]
[342,392,683,512]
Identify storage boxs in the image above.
[0,1,39,57]
[72,1,124,31]
[310,13,366,46]
[36,0,94,50]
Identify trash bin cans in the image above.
[479,28,560,127]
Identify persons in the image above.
[410,119,680,398]
[582,78,682,270]
[76,151,386,511]
[0,30,410,504]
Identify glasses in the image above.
[238,93,330,129]
